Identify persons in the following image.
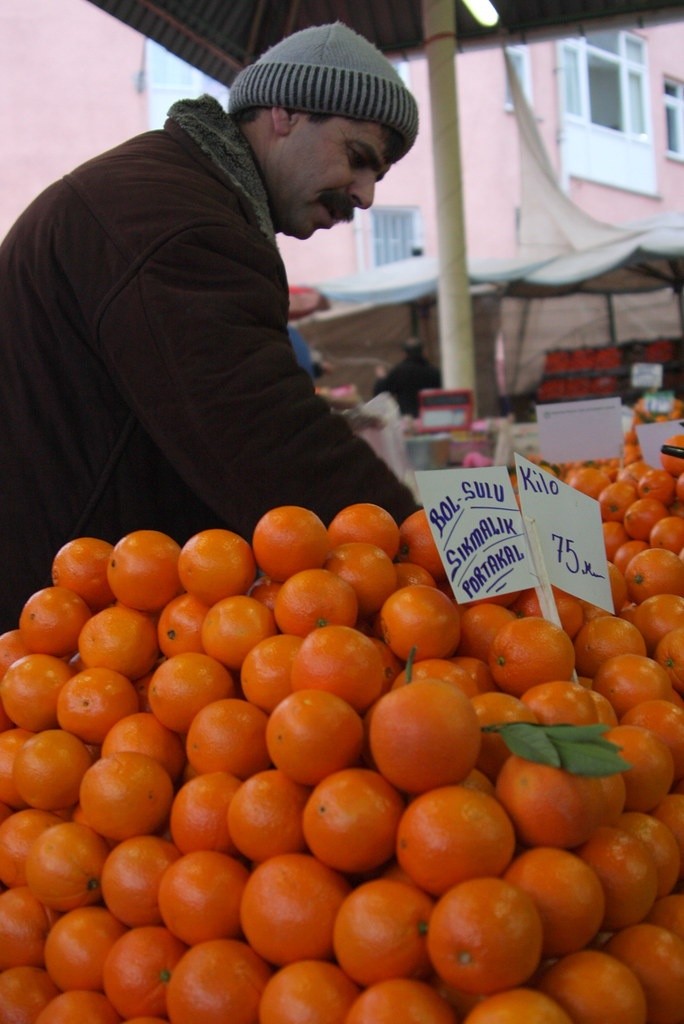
[288,323,336,381]
[0,20,425,636]
[373,338,443,417]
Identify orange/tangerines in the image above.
[0,394,684,1024]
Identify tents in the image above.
[287,209,684,417]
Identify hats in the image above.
[228,22,418,165]
